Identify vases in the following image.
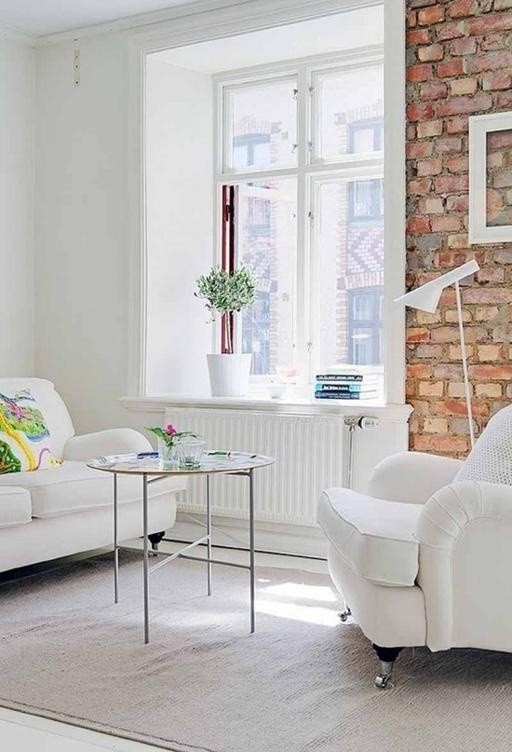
[159,446,180,471]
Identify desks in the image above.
[86,452,273,644]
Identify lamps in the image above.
[393,259,480,450]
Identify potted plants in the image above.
[194,264,256,397]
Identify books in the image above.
[314,374,379,400]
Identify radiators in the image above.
[163,407,380,529]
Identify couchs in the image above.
[0,376,187,574]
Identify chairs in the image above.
[318,402,512,692]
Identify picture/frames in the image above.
[468,111,512,244]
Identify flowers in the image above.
[145,424,200,452]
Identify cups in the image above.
[180,441,204,467]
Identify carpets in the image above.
[0,548,512,752]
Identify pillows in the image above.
[0,388,64,476]
